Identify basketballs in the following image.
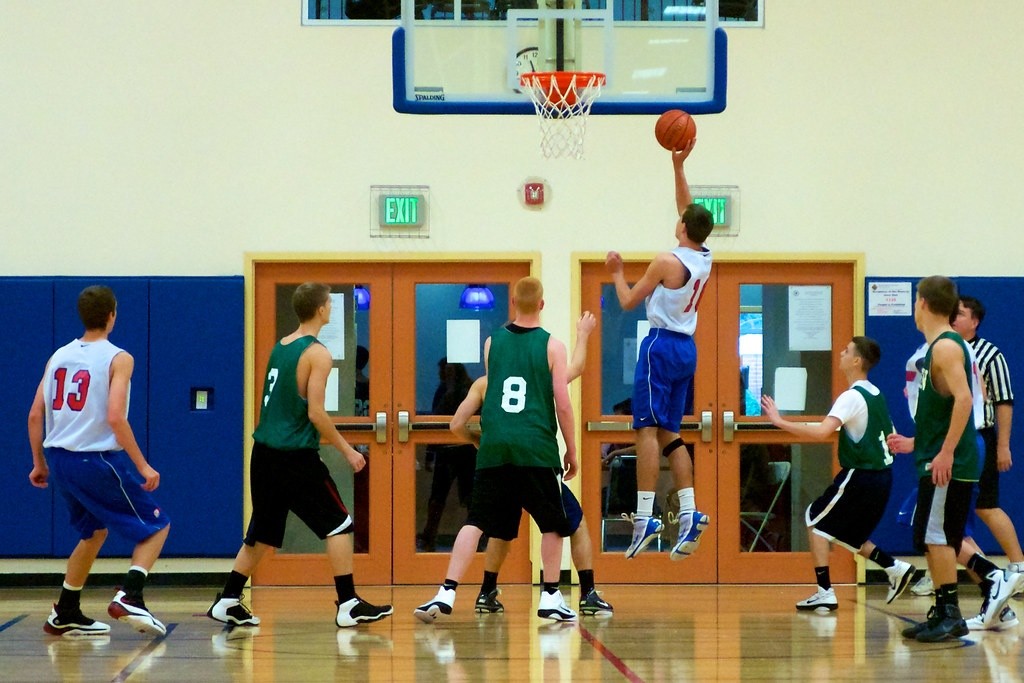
[655,109,697,152]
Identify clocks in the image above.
[513,47,539,93]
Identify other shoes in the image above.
[415,534,435,553]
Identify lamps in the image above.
[459,284,495,310]
[354,284,370,311]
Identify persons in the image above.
[412,277,583,624]
[886,276,1024,644]
[29,284,170,640]
[354,344,372,553]
[418,357,485,553]
[205,282,394,629]
[450,312,614,617]
[758,337,916,612]
[600,396,659,519]
[604,138,713,561]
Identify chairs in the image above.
[600,455,663,552]
[740,460,791,552]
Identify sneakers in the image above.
[621,512,665,559]
[797,612,836,637]
[414,630,456,664]
[959,630,1019,654]
[475,591,504,613]
[538,621,575,635]
[910,576,935,596]
[336,629,394,656]
[414,586,456,623]
[668,511,710,561]
[108,588,166,636]
[207,593,260,626]
[43,603,110,636]
[796,585,838,611]
[537,590,577,621]
[334,594,393,628]
[579,589,613,614]
[964,606,1018,630]
[884,559,916,605]
[980,569,1022,628]
[44,636,110,664]
[1003,567,1024,598]
[916,604,969,642]
[902,605,945,638]
[475,612,503,623]
